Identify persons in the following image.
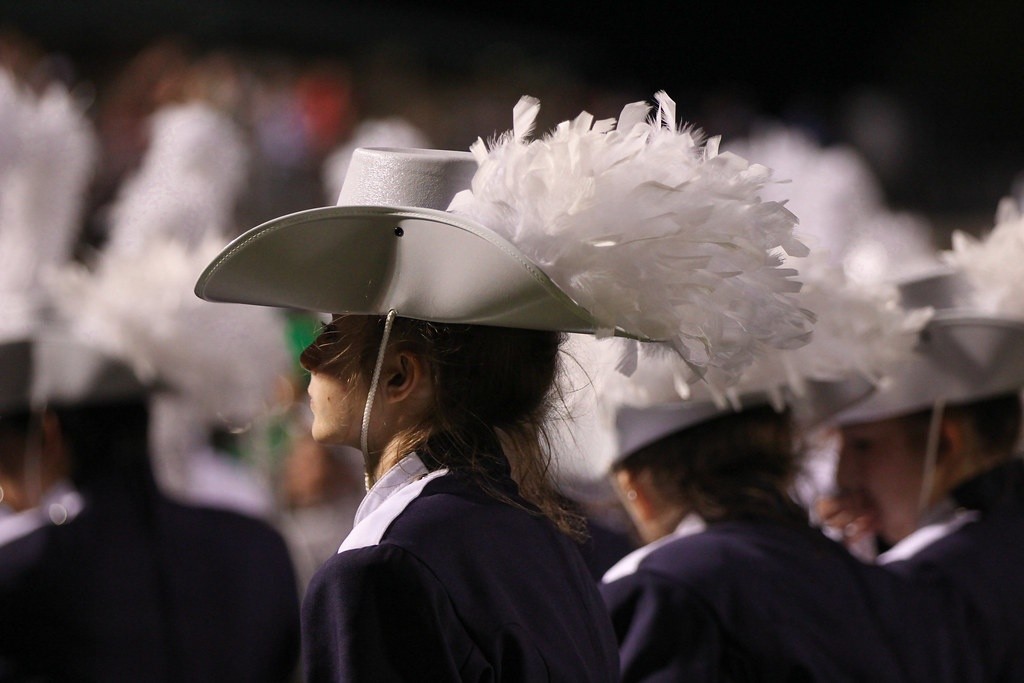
[770,320,1023,682]
[0,338,299,682]
[199,148,663,681]
[545,398,854,682]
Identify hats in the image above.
[194,144,674,345]
[1,67,1023,475]
